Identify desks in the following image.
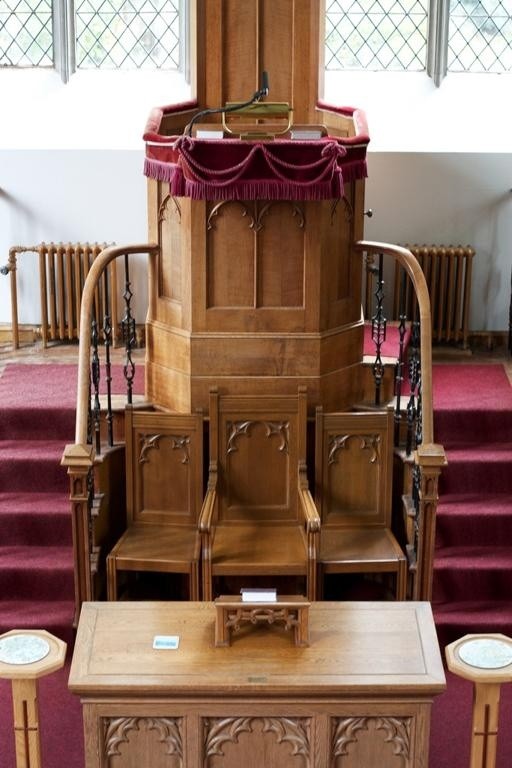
[64,600,450,768]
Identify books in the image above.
[240,588,278,602]
[196,129,225,140]
[288,127,323,141]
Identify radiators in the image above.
[360,238,495,355]
[0,237,145,352]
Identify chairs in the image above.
[103,400,205,603]
[196,381,324,601]
[308,397,410,601]
